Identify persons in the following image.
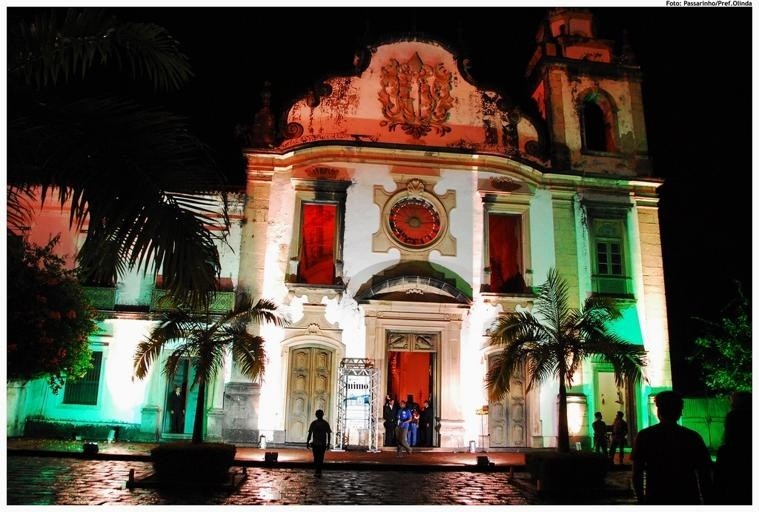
[307,410,332,477]
[170,387,185,433]
[384,398,432,457]
[593,412,608,457]
[631,392,752,504]
[609,412,628,464]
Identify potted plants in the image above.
[131,258,279,483]
[483,258,648,480]
